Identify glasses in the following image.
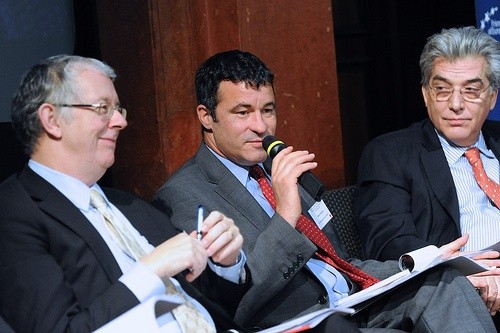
[37,101,126,120]
[428,79,492,103]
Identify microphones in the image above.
[262,135,326,201]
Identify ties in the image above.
[464,146,500,209]
[90,189,214,333]
[248,164,382,290]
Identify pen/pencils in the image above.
[189,205,203,272]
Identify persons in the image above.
[0,54,253,333]
[149,50,500,333]
[354,25,500,333]
